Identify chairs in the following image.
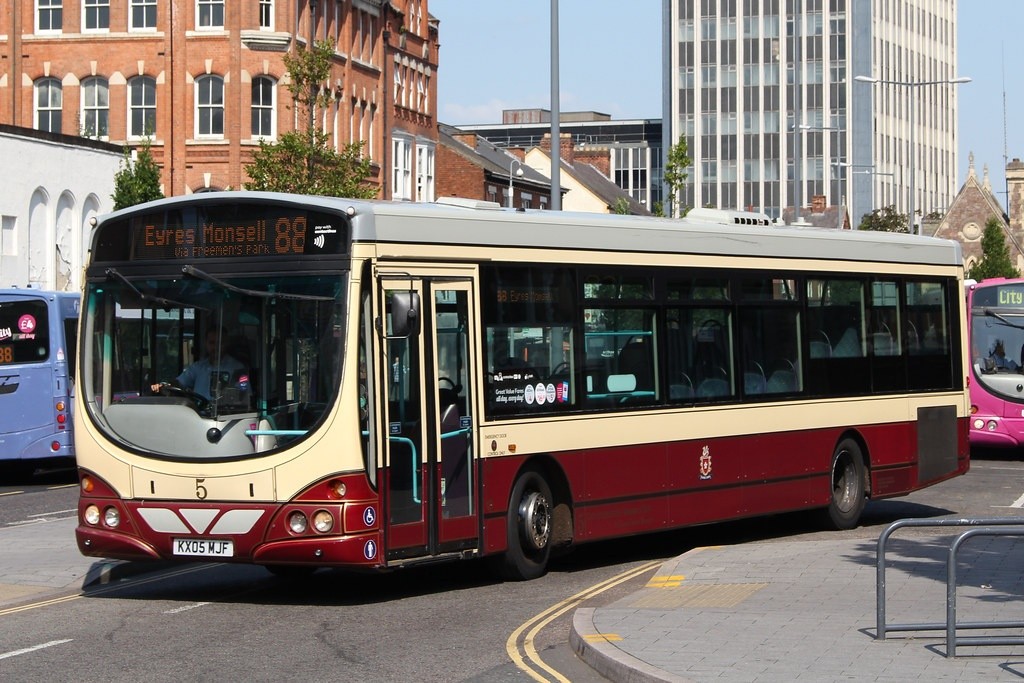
[493,318,947,415]
[228,334,258,407]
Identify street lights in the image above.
[509,160,524,208]
[830,161,877,212]
[854,75,973,235]
[792,124,843,229]
[866,164,897,212]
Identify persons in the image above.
[989,343,1016,370]
[393,357,406,401]
[150,324,252,415]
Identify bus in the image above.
[72,189,972,580]
[0,286,194,483]
[966,277,1024,448]
